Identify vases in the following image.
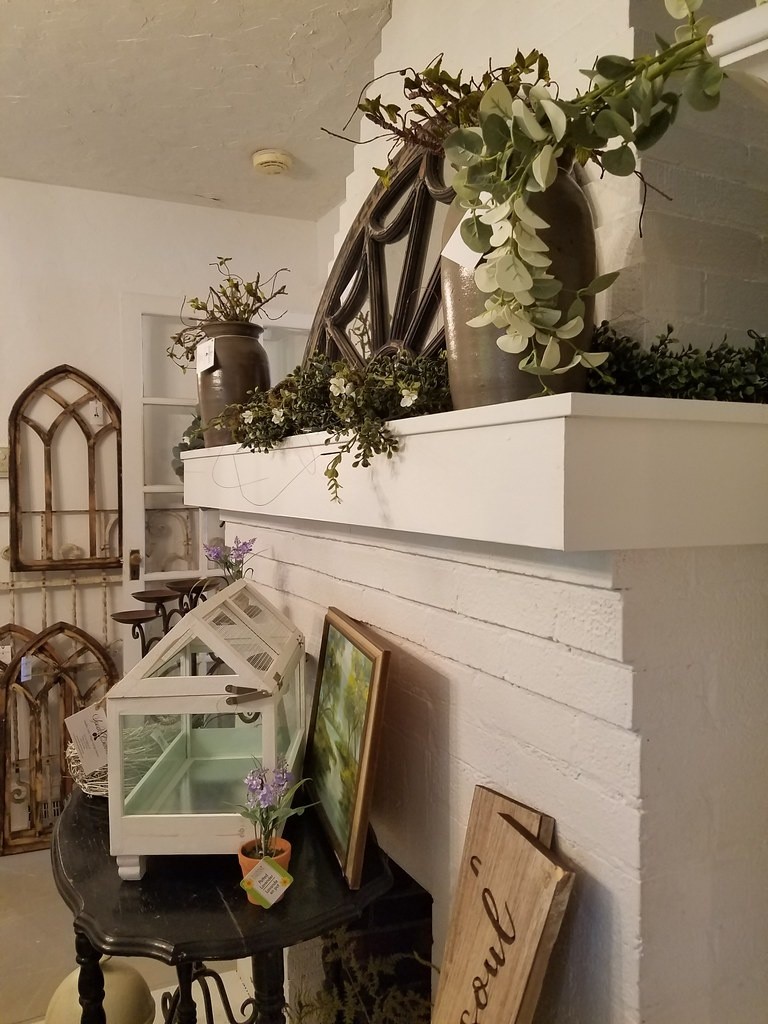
[238,836,292,906]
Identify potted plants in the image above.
[165,256,291,448]
[321,0,731,412]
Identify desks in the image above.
[49,787,395,1024]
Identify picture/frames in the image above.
[303,606,391,890]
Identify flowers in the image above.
[188,535,269,607]
[220,753,322,860]
